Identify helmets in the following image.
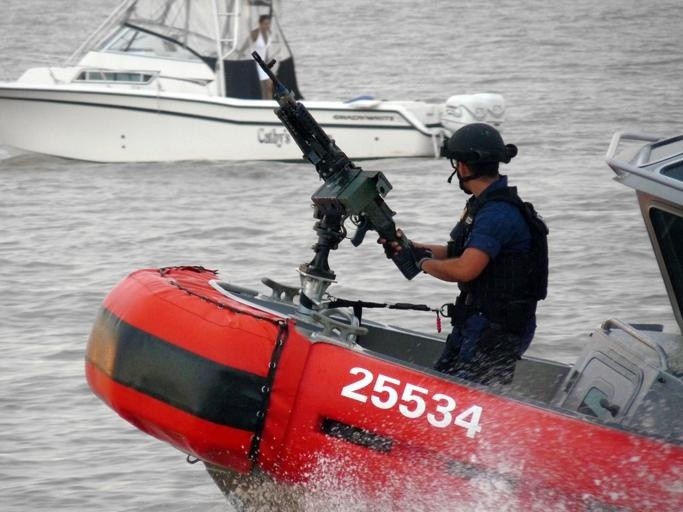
[438,120,518,177]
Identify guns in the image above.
[251,51,423,280]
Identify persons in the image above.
[377,123,550,393]
[238,13,279,99]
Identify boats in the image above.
[1,1,513,169]
[79,133,683,511]
